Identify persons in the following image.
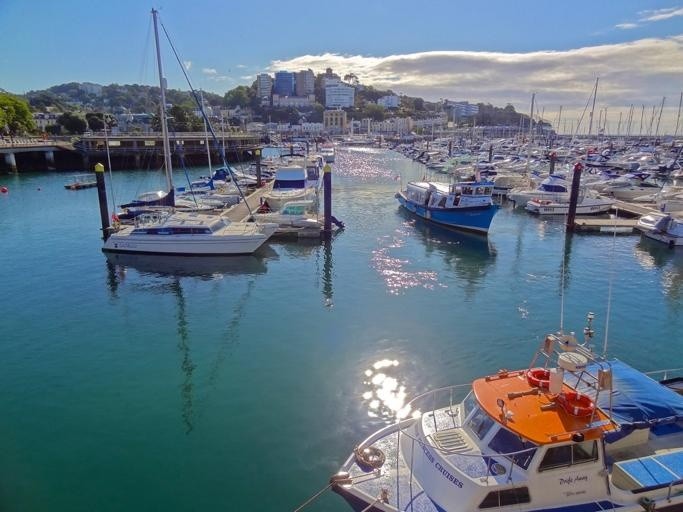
[425,186,490,208]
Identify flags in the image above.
[394,175,401,182]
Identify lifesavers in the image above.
[527,368,551,388]
[560,392,595,416]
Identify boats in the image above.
[329,313,683,511]
